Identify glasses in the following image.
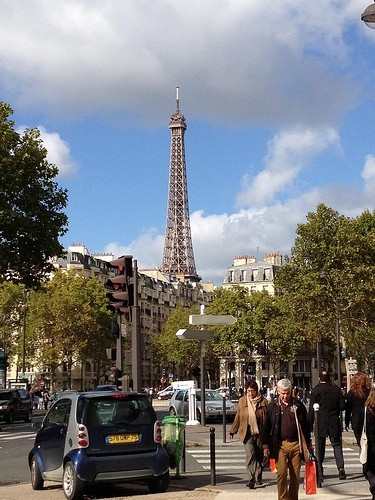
[247,388,256,393]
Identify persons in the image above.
[228,371,375,500]
[29,389,56,410]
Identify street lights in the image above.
[320,268,341,392]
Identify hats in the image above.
[319,371,330,378]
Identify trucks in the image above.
[157,379,198,400]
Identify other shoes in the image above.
[246,481,255,488]
[339,469,346,479]
[256,479,263,485]
[318,469,323,479]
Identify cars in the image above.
[50,392,81,412]
[0,388,33,423]
[25,391,172,500]
[95,385,118,392]
[168,389,237,422]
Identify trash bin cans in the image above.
[162,416,187,469]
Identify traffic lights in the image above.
[105,254,133,314]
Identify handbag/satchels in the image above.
[304,452,317,494]
[359,432,368,464]
